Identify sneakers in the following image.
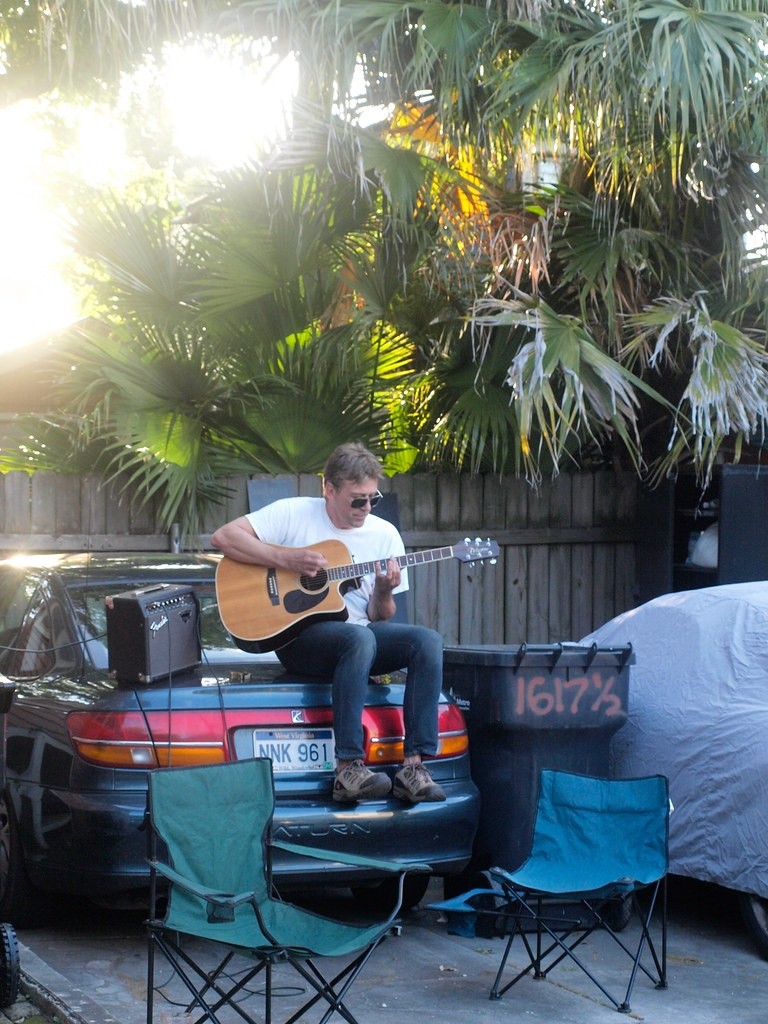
[392,761,446,803]
[333,759,392,801]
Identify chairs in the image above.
[143,757,434,1024]
[423,767,674,1015]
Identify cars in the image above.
[0,552,482,929]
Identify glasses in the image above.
[330,482,383,509]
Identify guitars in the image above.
[213,535,503,654]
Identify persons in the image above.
[208,441,448,803]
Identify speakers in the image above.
[104,583,201,685]
